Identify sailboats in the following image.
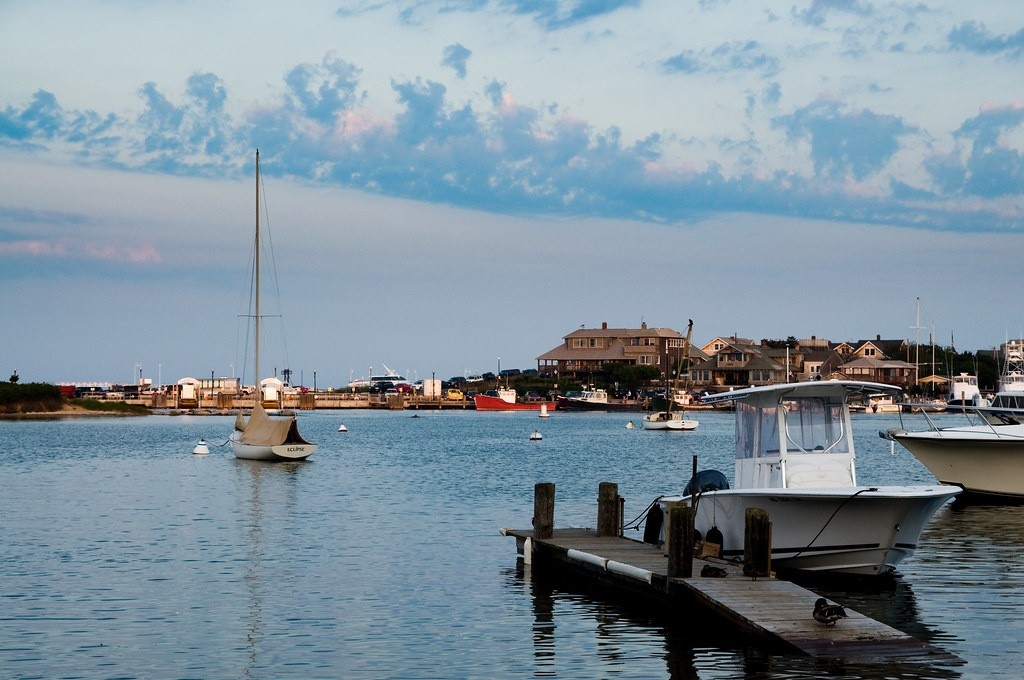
[643,319,699,430]
[226,147,319,463]
[900,297,992,413]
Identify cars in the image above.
[526,390,541,400]
[410,379,423,391]
[395,383,410,392]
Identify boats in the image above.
[879,402,1024,505]
[657,377,961,579]
[557,386,643,411]
[975,329,1024,424]
[472,393,556,411]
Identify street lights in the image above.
[432,370,435,401]
[497,357,501,375]
[211,369,215,400]
[274,367,277,378]
[313,370,318,393]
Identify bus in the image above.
[372,376,408,384]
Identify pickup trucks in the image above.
[384,390,399,401]
[447,388,464,400]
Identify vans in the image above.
[370,382,394,394]
